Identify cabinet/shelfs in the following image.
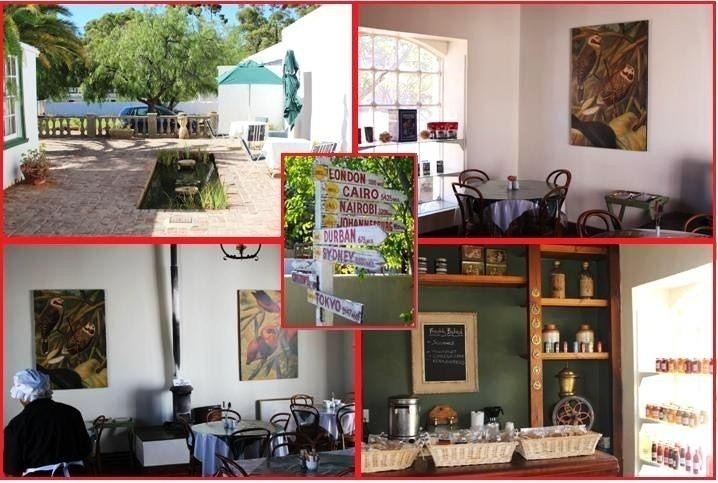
[528,244,624,477]
[361,434,620,477]
[415,243,528,286]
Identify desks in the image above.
[588,227,712,237]
[457,179,566,200]
[247,451,354,478]
[290,399,355,415]
[263,137,314,167]
[188,419,289,437]
[230,120,269,150]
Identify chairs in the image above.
[336,404,355,451]
[268,432,312,458]
[537,186,569,237]
[312,141,338,153]
[340,392,355,404]
[253,116,269,123]
[452,182,491,239]
[546,168,572,191]
[459,168,490,187]
[246,124,266,149]
[239,136,267,177]
[683,212,713,237]
[205,119,230,149]
[577,209,624,237]
[289,404,335,453]
[269,412,292,432]
[229,427,271,461]
[291,394,314,407]
[213,452,249,477]
[178,413,195,456]
[207,408,242,423]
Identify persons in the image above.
[4,368,91,477]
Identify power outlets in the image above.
[363,409,370,423]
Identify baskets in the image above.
[507,426,602,460]
[426,433,518,467]
[362,439,423,473]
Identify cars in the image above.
[118,106,196,132]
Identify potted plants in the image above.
[19,142,52,186]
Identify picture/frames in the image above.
[30,289,108,391]
[569,19,650,152]
[236,289,299,382]
[410,312,479,395]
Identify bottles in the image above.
[646,401,708,425]
[655,357,714,375]
[554,342,559,352]
[574,341,578,354]
[580,341,585,353]
[437,160,444,174]
[505,421,514,442]
[435,258,447,275]
[588,342,592,352]
[575,323,594,351]
[693,448,699,475]
[564,342,568,353]
[651,440,684,469]
[541,324,560,352]
[423,162,430,176]
[550,260,565,298]
[486,417,499,442]
[597,342,602,354]
[330,392,335,407]
[579,260,594,297]
[418,257,427,275]
[685,447,692,472]
[545,341,549,353]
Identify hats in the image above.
[10,369,53,401]
